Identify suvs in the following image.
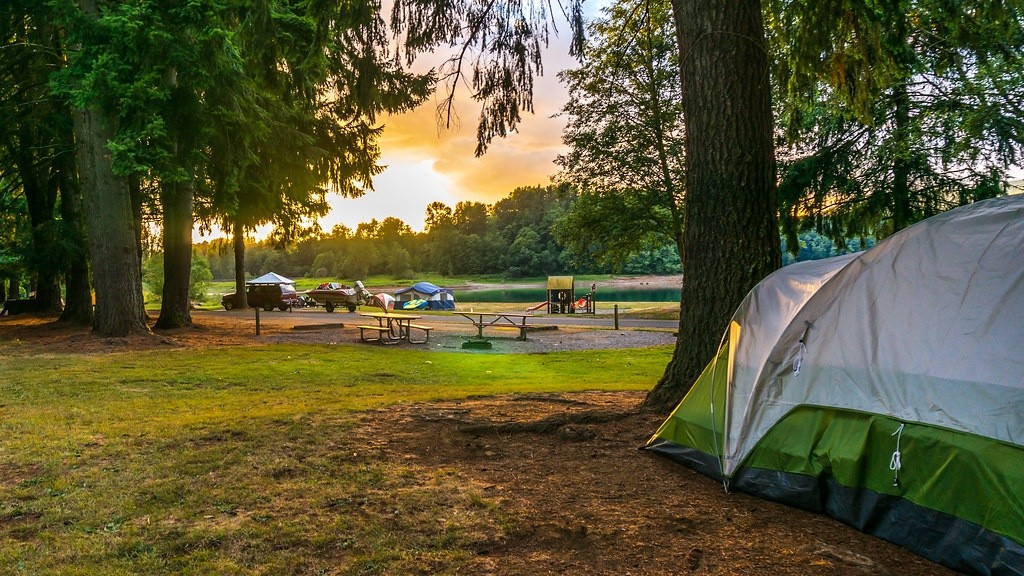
[220,283,296,312]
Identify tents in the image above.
[246,272,296,292]
[393,282,463,312]
[638,193,1024,576]
[367,293,395,310]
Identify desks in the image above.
[452,311,534,341]
[360,313,429,345]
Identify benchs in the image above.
[473,323,535,329]
[357,326,391,331]
[397,323,435,330]
